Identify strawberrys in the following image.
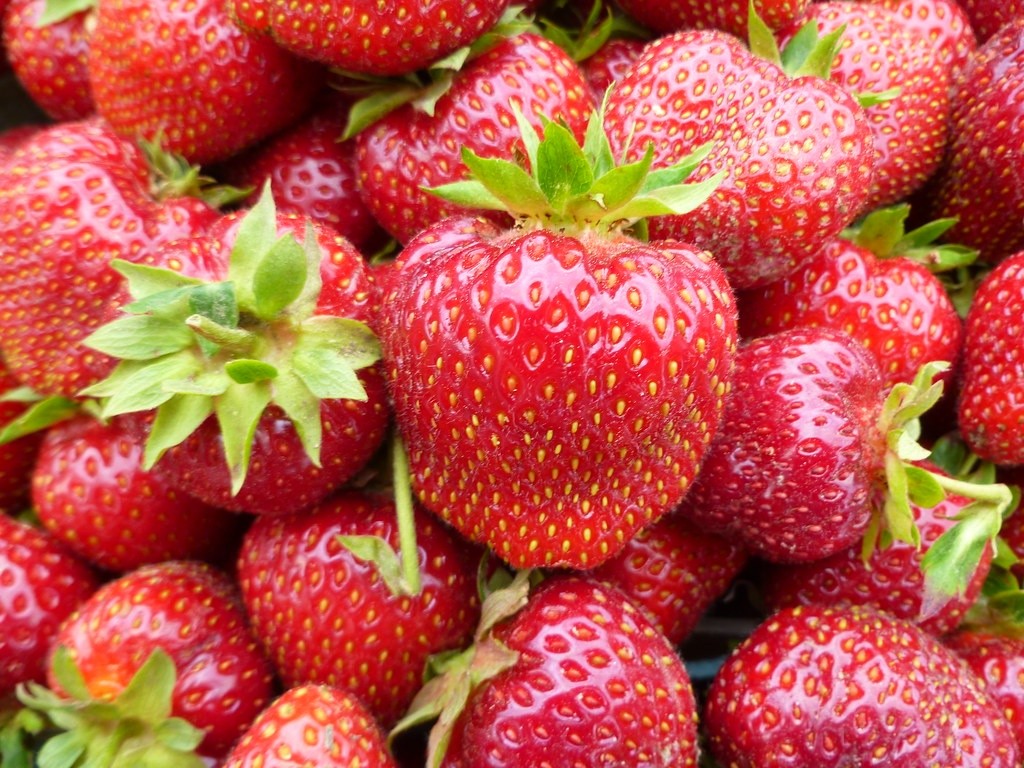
[0,0,1024,768]
